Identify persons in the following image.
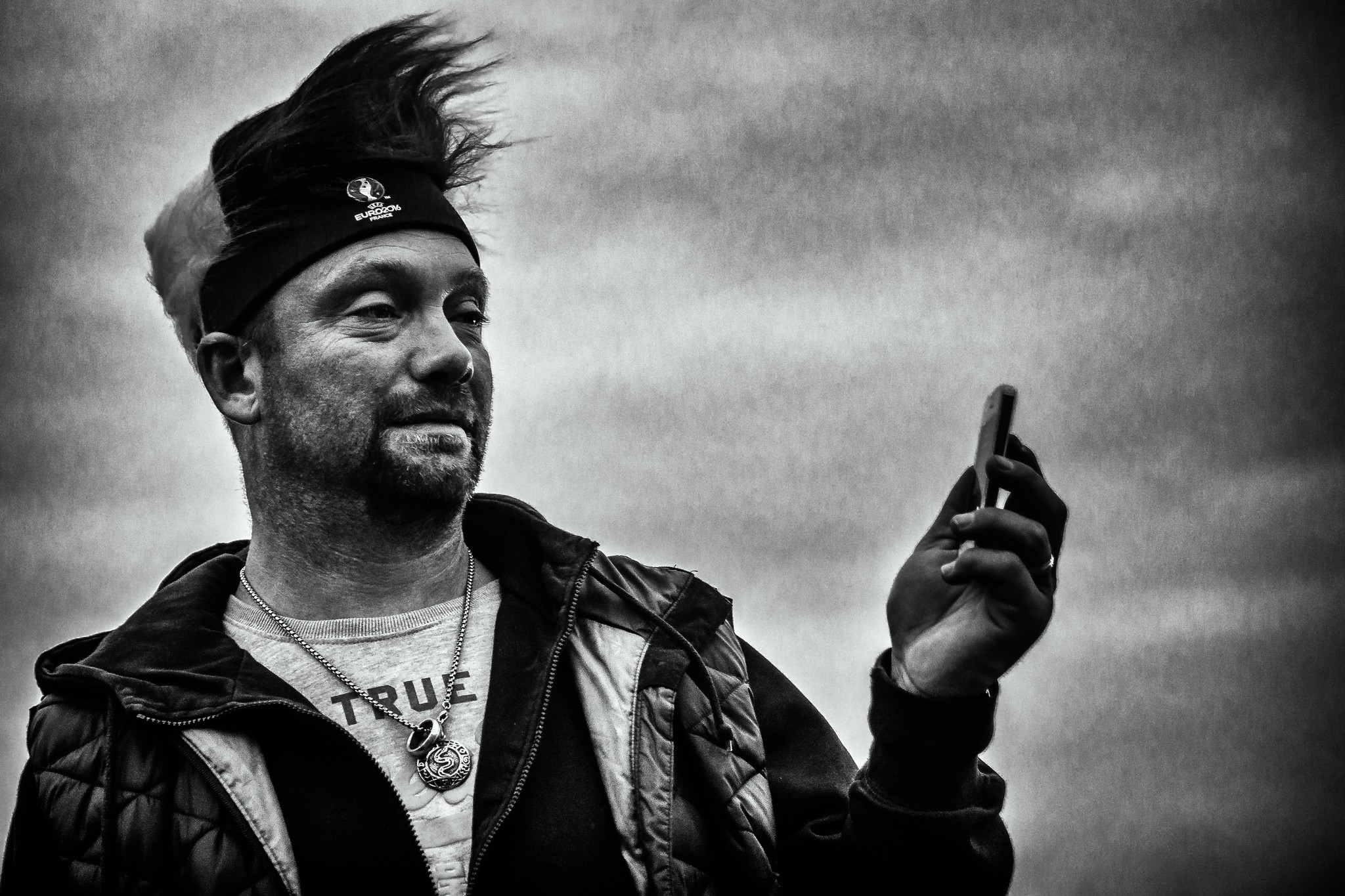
[0,16,1066,896]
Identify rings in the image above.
[1040,555,1054,572]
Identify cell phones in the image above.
[957,384,1018,577]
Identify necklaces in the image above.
[240,549,474,792]
